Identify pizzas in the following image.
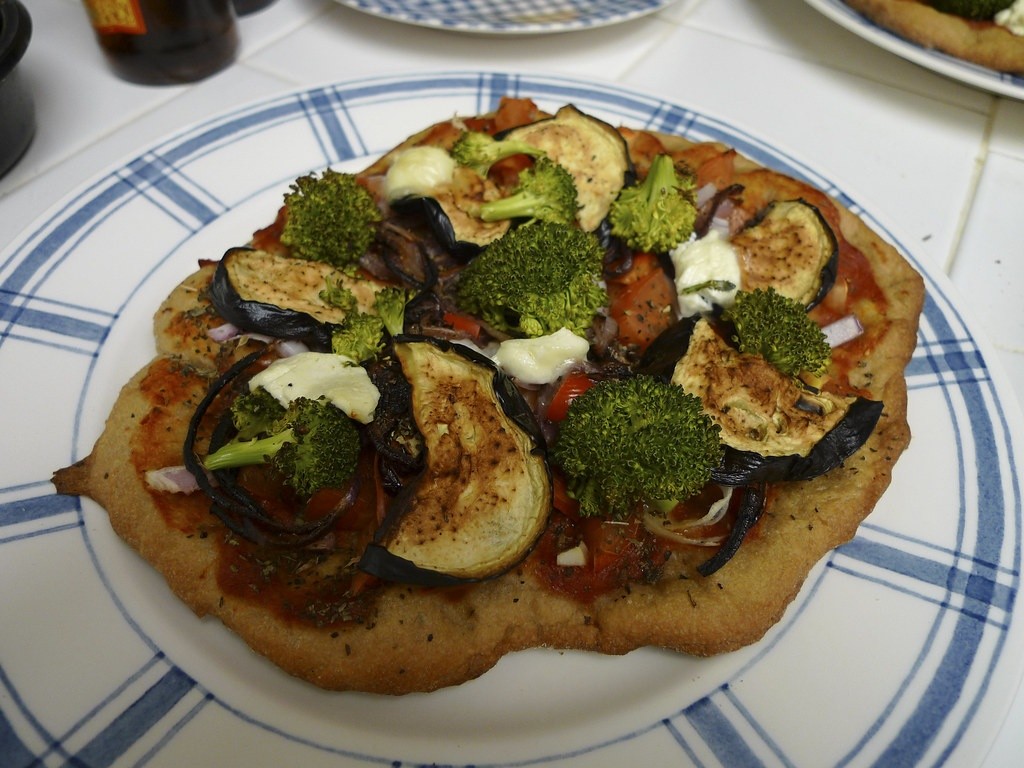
[846,0,1024,78]
[50,98,928,696]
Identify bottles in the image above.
[234,0,279,16]
[81,0,242,86]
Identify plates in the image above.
[337,0,672,35]
[0,72,1024,768]
[806,0,1024,101]
